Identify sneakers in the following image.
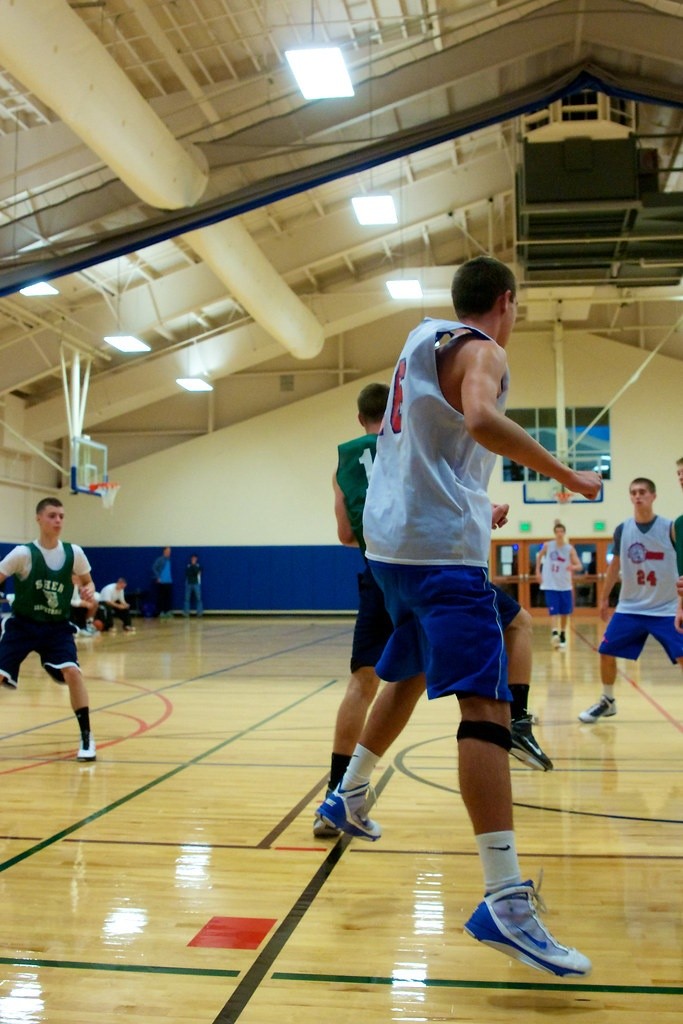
[77,732,97,761]
[509,711,553,774]
[79,763,95,777]
[551,635,567,649]
[578,695,617,722]
[318,781,382,841]
[580,723,618,750]
[462,869,591,979]
[312,816,340,840]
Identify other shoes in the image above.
[109,628,117,631]
[124,625,136,630]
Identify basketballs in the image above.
[93,620,104,632]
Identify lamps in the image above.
[351,24,400,227]
[21,278,61,298]
[174,316,214,393]
[386,229,424,302]
[283,0,355,101]
[104,262,154,353]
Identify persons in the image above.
[319,256,591,977]
[0,497,95,761]
[586,547,597,606]
[578,476,679,723]
[535,524,583,649]
[311,382,551,838]
[151,545,175,624]
[71,577,101,636]
[97,578,132,633]
[512,554,519,603]
[670,459,683,634]
[183,555,205,620]
